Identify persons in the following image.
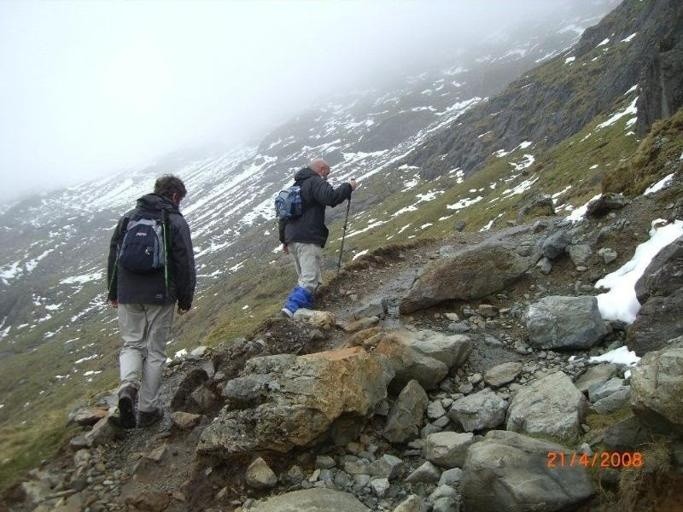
[107,176,197,429]
[277,160,356,319]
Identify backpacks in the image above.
[117,208,167,274]
[276,182,303,222]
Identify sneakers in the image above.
[138,409,164,428]
[282,307,294,319]
[117,390,135,427]
